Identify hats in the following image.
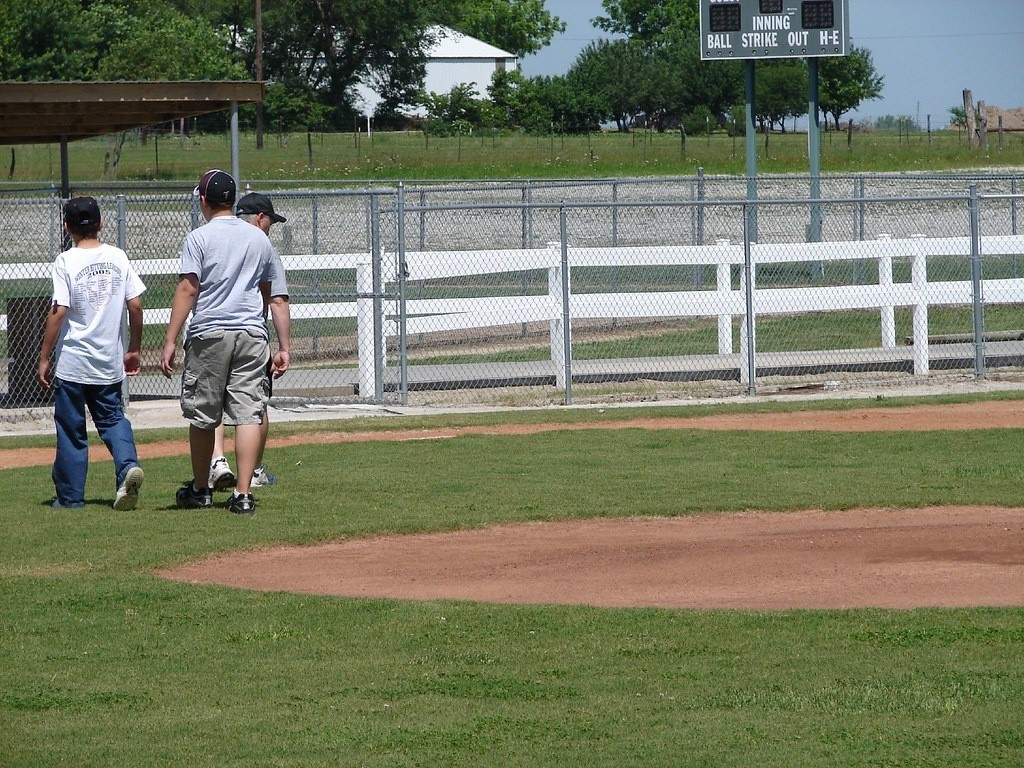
[194,168,236,203]
[236,193,287,222]
[65,197,100,225]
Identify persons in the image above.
[38,196,147,511]
[161,168,291,514]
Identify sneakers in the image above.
[227,493,254,514]
[209,456,234,490]
[250,467,275,488]
[176,479,212,509]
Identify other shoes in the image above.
[113,467,144,512]
[52,499,66,509]
